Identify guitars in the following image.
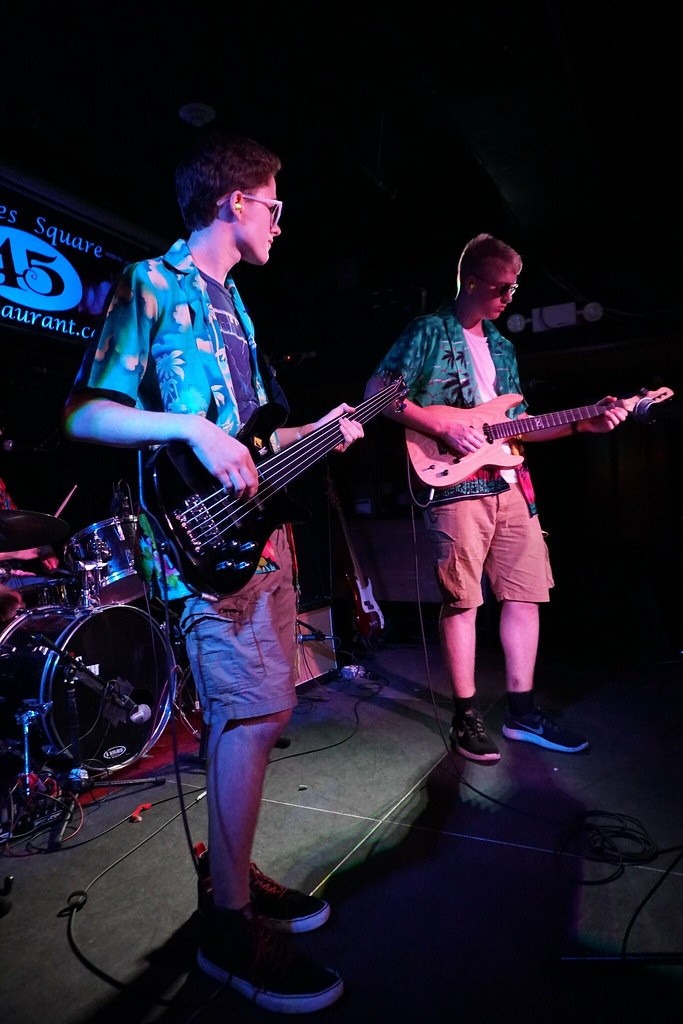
[327,475,388,633]
[137,371,407,600]
[401,385,676,491]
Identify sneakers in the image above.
[195,850,330,932]
[448,707,501,761]
[199,921,343,1014]
[502,703,590,751]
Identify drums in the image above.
[60,514,149,611]
[12,575,83,610]
[0,603,177,781]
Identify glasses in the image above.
[470,272,520,297]
[215,190,285,228]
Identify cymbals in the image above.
[0,506,71,558]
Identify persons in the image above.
[0,583,22,621]
[0,477,59,577]
[63,136,365,1015]
[362,234,628,761]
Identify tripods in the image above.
[31,634,166,850]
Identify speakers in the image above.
[294,595,340,692]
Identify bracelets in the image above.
[571,422,577,434]
[296,426,304,440]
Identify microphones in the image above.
[3,439,43,454]
[111,479,125,513]
[130,703,152,724]
[297,634,339,643]
